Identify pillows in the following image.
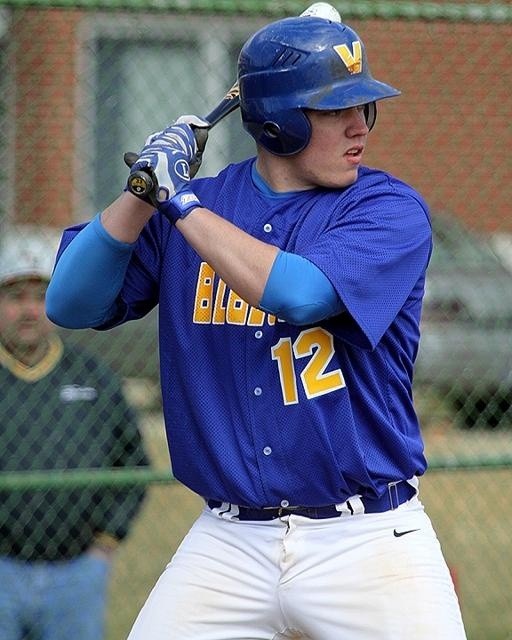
[235,14,403,158]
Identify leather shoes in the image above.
[120,113,213,227]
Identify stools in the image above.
[202,477,418,524]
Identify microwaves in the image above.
[128,3,342,195]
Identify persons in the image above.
[1,225,152,639]
[42,19,468,639]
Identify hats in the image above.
[412,219,510,427]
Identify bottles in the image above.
[0,234,56,288]
